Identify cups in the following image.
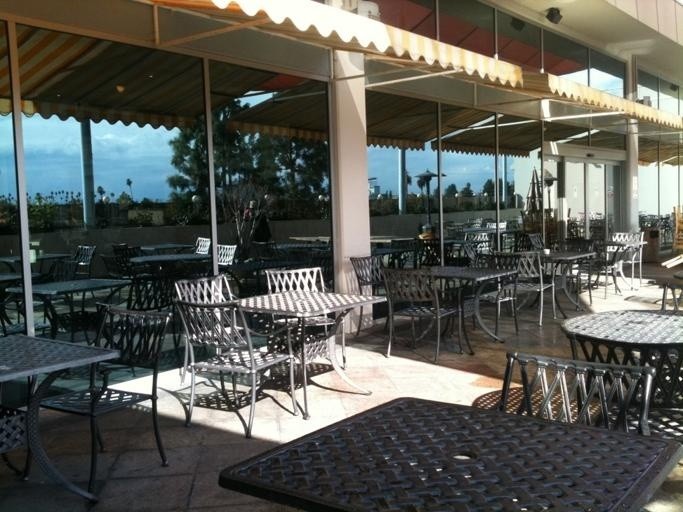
[544,249,550,254]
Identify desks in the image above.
[2,334,125,510]
[0,251,73,286]
[220,398,683,512]
[5,277,134,372]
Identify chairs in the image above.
[48,215,532,349]
[22,227,683,494]
